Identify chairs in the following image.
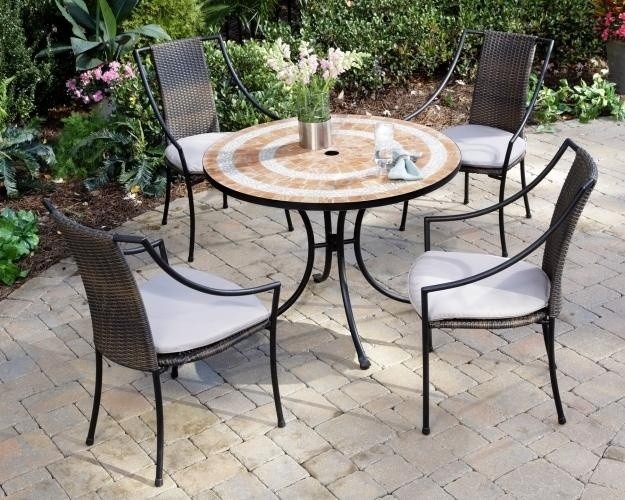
[399,28,554,258]
[41,197,285,487]
[407,138,599,435]
[132,33,295,262]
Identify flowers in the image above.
[267,35,373,121]
[65,60,133,124]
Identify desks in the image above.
[202,112,462,371]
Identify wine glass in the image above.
[373,121,394,177]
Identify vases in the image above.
[300,113,333,151]
